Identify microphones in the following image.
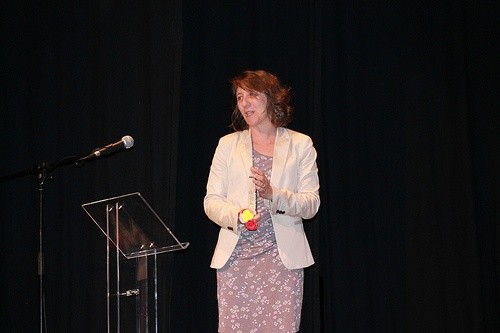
[78,135,134,162]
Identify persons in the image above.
[202,70,321,333]
